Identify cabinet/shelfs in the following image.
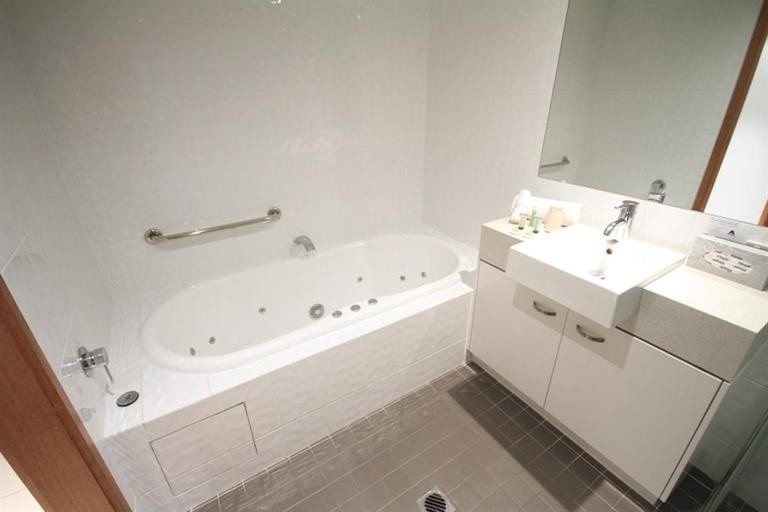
[464,211,768,512]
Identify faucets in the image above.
[647,180,666,203]
[605,200,641,242]
[293,235,316,257]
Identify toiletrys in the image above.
[515,203,564,234]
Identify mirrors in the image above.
[537,0,766,225]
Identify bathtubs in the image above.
[144,232,468,374]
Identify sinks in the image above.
[507,223,687,329]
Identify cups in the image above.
[544,206,565,234]
